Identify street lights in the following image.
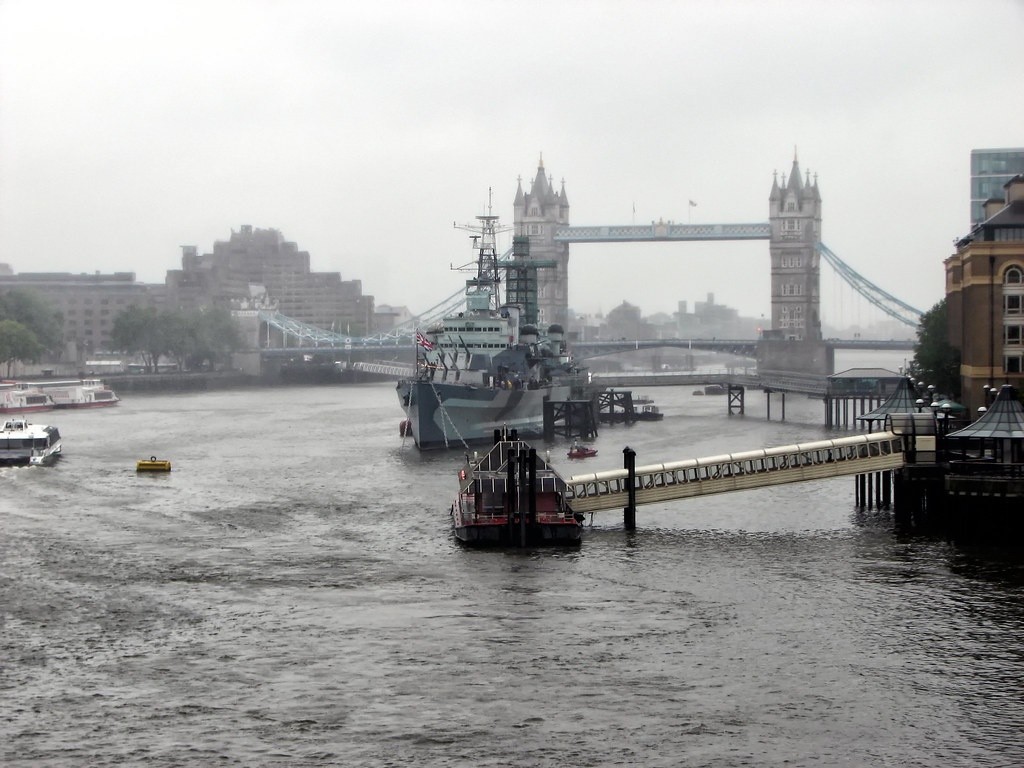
[928,384,935,401]
[913,397,925,414]
[940,402,952,460]
[977,405,987,457]
[930,400,941,418]
[918,381,925,396]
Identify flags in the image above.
[689,201,697,206]
[416,332,432,352]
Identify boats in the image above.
[448,421,590,549]
[566,445,600,458]
[0,414,64,467]
[135,454,172,473]
[398,418,412,436]
[394,187,592,450]
[18,376,121,410]
[640,402,660,414]
[0,383,58,413]
